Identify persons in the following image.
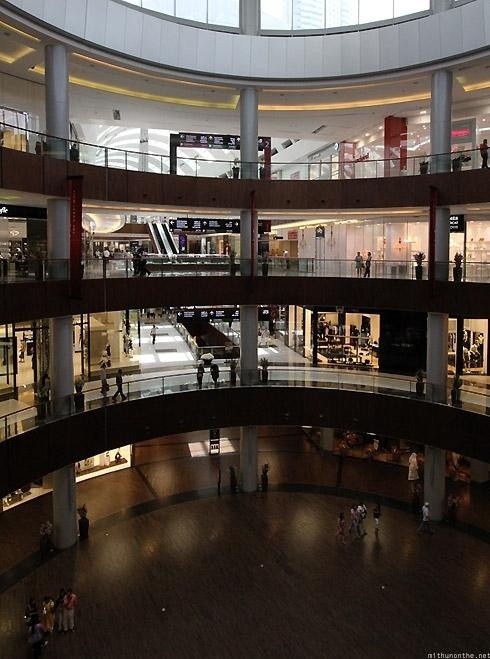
[100,364,108,398]
[24,588,79,657]
[445,452,473,525]
[196,364,204,390]
[259,468,269,496]
[334,501,384,547]
[223,239,234,258]
[210,361,220,383]
[227,464,238,496]
[95,245,152,278]
[415,502,437,536]
[283,249,291,271]
[150,324,159,345]
[355,252,372,277]
[480,139,489,169]
[407,452,424,497]
[269,310,277,336]
[18,340,26,363]
[111,369,128,401]
